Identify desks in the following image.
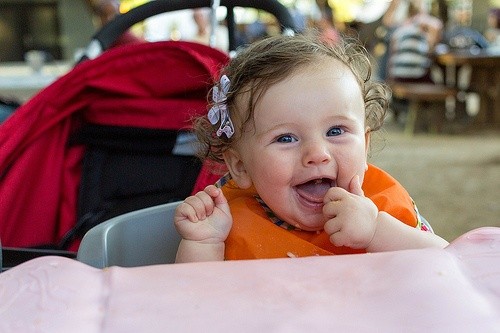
[439,51,500,128]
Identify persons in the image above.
[193,1,500,113]
[174,30,450,269]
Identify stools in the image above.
[391,87,453,135]
